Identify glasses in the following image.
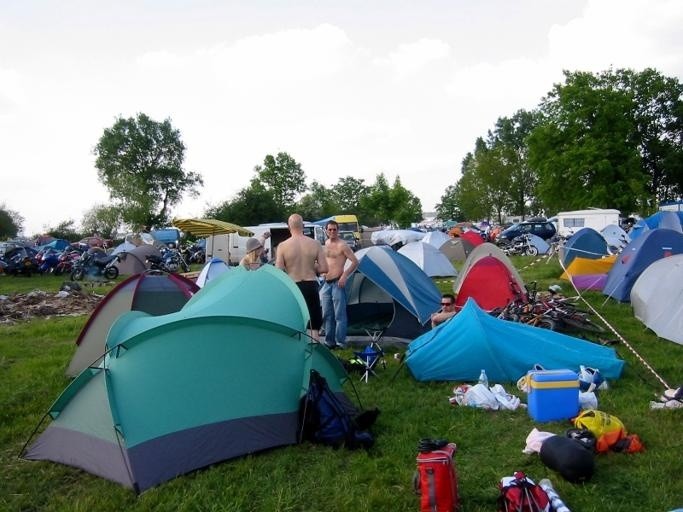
[439,301,453,306]
[325,227,339,232]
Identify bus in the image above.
[314,214,363,253]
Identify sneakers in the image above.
[324,341,348,352]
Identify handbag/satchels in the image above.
[413,439,462,512]
[575,366,611,391]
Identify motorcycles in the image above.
[497,232,538,257]
[0,240,204,282]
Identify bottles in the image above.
[478,369,488,390]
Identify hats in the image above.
[246,238,263,254]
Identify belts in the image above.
[322,272,344,285]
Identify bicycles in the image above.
[483,280,606,334]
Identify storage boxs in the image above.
[524,368,581,423]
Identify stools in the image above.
[351,345,383,385]
[365,324,387,361]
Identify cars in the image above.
[408,214,556,242]
[337,231,360,252]
